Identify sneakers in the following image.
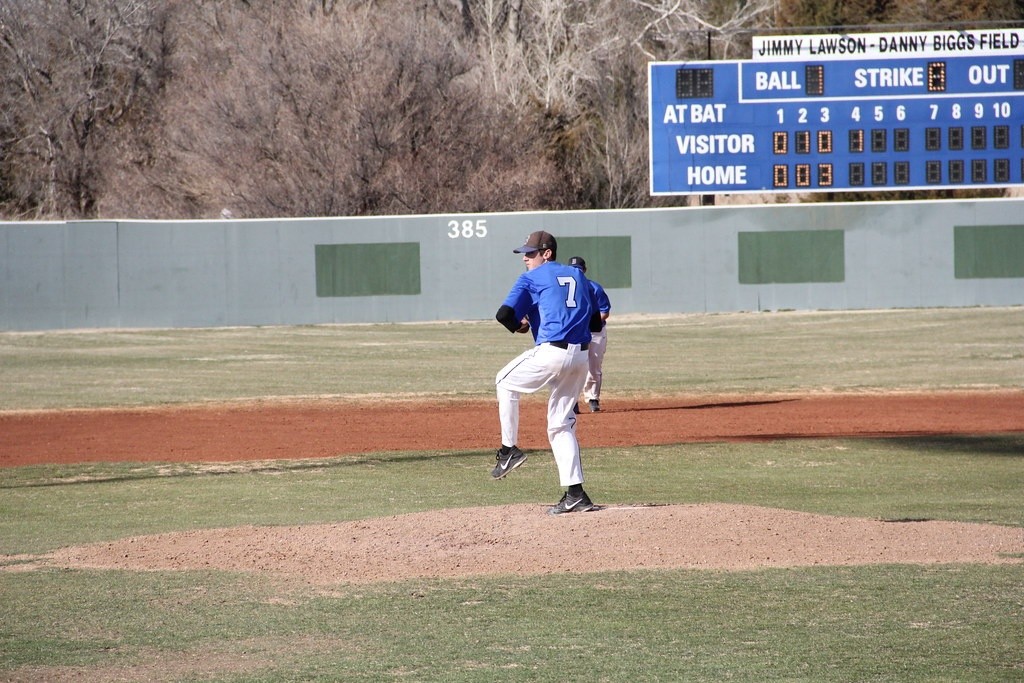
[546,491,594,515]
[491,445,528,480]
[589,399,601,412]
[573,402,580,414]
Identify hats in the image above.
[513,231,557,253]
[568,257,585,268]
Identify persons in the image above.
[568,256,611,413]
[492,230,602,514]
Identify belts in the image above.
[602,321,606,327]
[539,341,588,352]
